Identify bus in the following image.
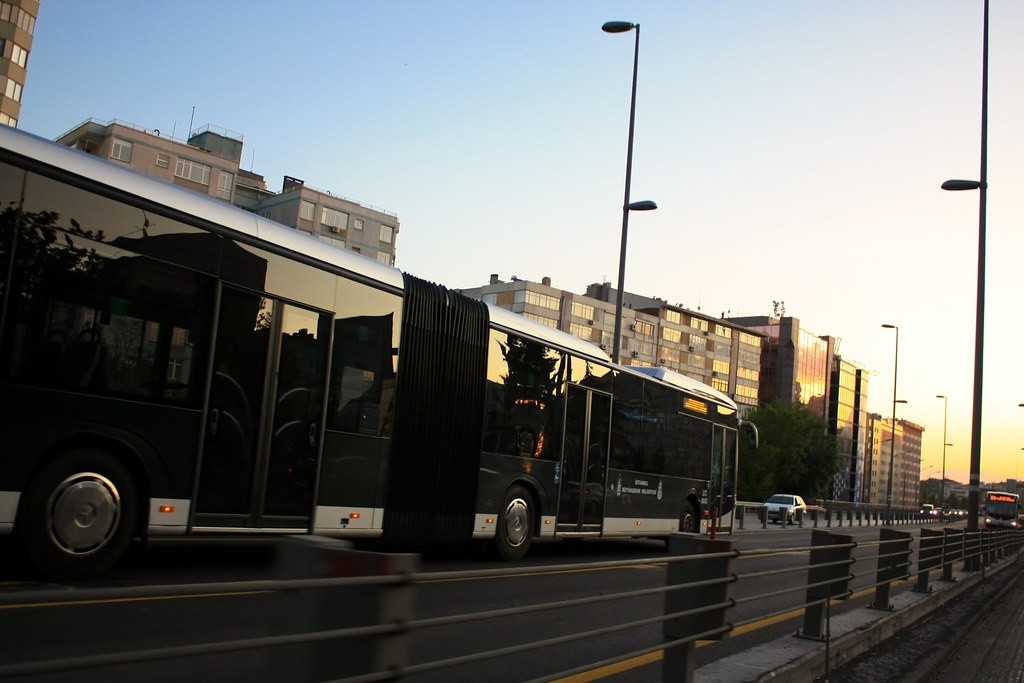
[0,126,760,582]
[983,491,1022,530]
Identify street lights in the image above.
[943,179,989,570]
[602,20,659,362]
[881,322,909,521]
[936,395,954,517]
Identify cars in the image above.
[759,493,807,525]
[919,504,968,519]
